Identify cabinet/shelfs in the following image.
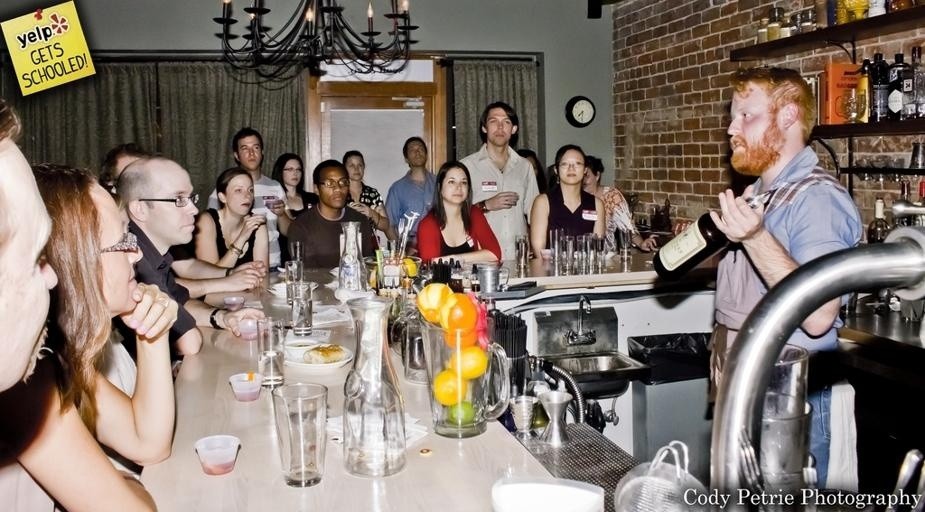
[730,0,925,345]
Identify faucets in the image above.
[567,294,596,346]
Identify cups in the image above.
[855,158,905,181]
[489,473,608,512]
[722,345,816,511]
[193,434,240,477]
[477,264,512,293]
[270,381,331,485]
[262,196,277,208]
[511,226,638,274]
[842,89,866,123]
[218,234,512,440]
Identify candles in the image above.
[222,0,231,18]
[367,2,374,32]
[305,7,315,34]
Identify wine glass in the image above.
[509,395,536,441]
[536,390,575,445]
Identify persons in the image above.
[1,101,658,512]
[713,68,866,495]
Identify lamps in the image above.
[212,0,419,90]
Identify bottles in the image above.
[867,178,924,246]
[910,141,925,171]
[653,190,771,283]
[857,45,925,125]
[756,0,923,43]
[843,286,925,325]
[336,295,405,479]
[336,220,367,301]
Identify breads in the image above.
[301,344,345,364]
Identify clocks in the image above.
[566,96,596,128]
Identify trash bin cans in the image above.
[627,333,714,486]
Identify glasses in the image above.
[99,232,138,252]
[139,193,199,207]
[319,180,350,187]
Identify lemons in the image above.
[413,283,489,426]
[402,257,418,278]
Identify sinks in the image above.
[537,351,651,401]
